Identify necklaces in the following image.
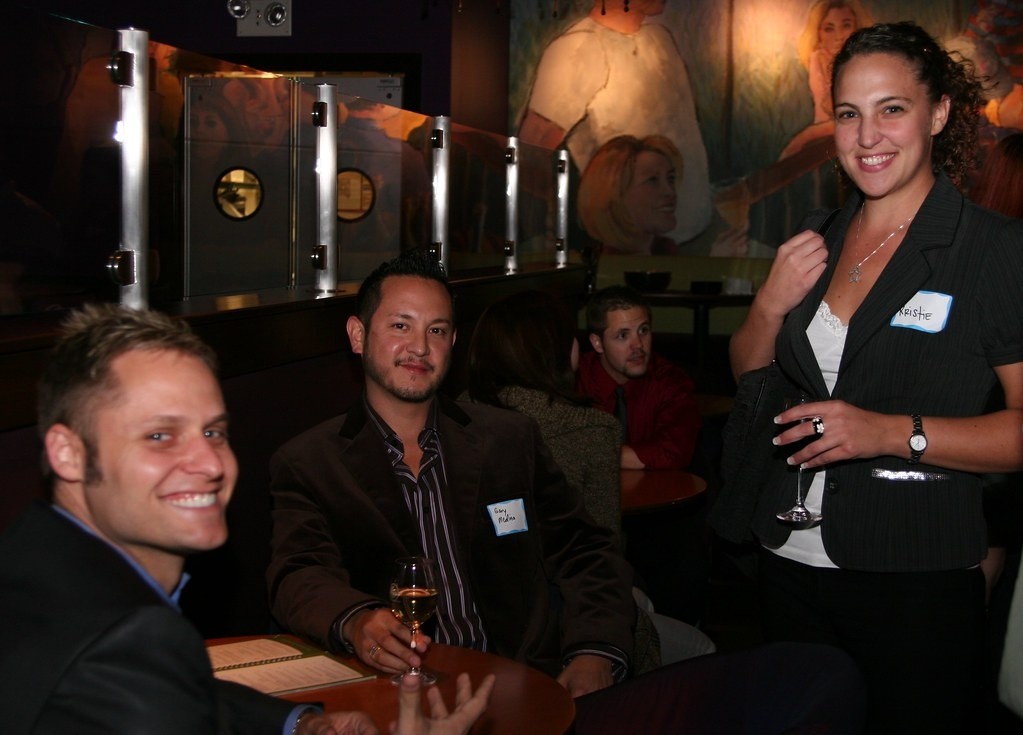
[848,202,915,282]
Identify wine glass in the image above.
[389,556,437,687]
[776,395,824,524]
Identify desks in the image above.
[583,285,758,373]
[683,390,737,422]
[617,466,709,516]
[183,631,574,735]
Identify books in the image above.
[205,638,376,697]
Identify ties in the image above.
[611,386,627,445]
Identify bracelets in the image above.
[292,712,303,735]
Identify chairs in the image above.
[496,381,629,539]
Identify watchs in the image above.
[909,414,928,463]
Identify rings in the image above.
[370,646,381,655]
[813,418,824,433]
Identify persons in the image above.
[971,132,1023,615]
[470,282,655,613]
[577,286,704,629]
[0,305,495,734]
[271,257,637,698]
[705,22,1023,735]
